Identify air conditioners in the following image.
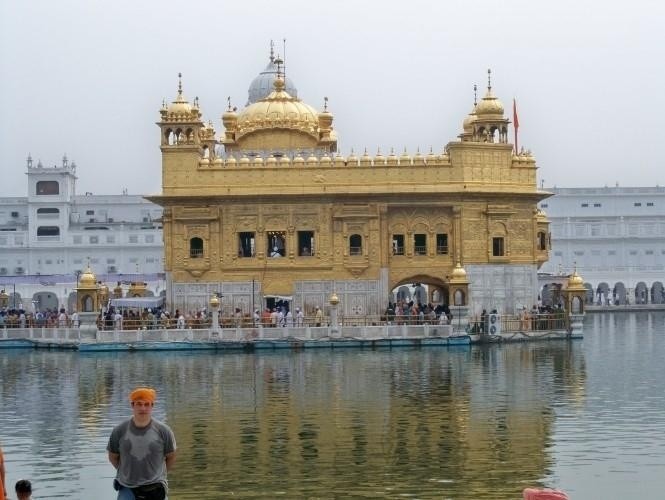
[483,314,502,326]
[483,323,502,335]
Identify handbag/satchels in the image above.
[131,483,165,500]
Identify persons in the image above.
[301,247,312,256]
[105,386,177,499]
[315,305,322,327]
[379,300,452,325]
[530,302,566,330]
[270,246,281,257]
[0,448,8,499]
[14,479,31,500]
[254,306,304,328]
[187,306,241,327]
[95,306,185,330]
[2,305,80,327]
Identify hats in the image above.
[130,389,156,402]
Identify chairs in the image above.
[1,310,283,331]
